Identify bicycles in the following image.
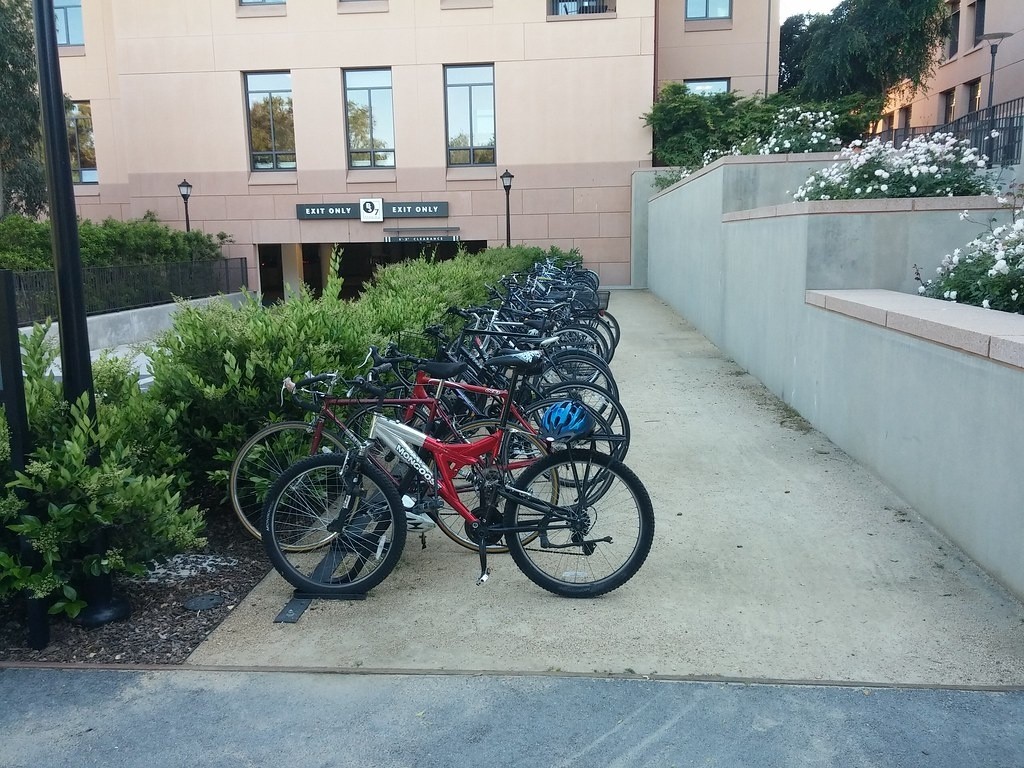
[229,253,655,600]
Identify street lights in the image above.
[499,167,515,248]
[177,178,192,233]
[976,30,1015,118]
